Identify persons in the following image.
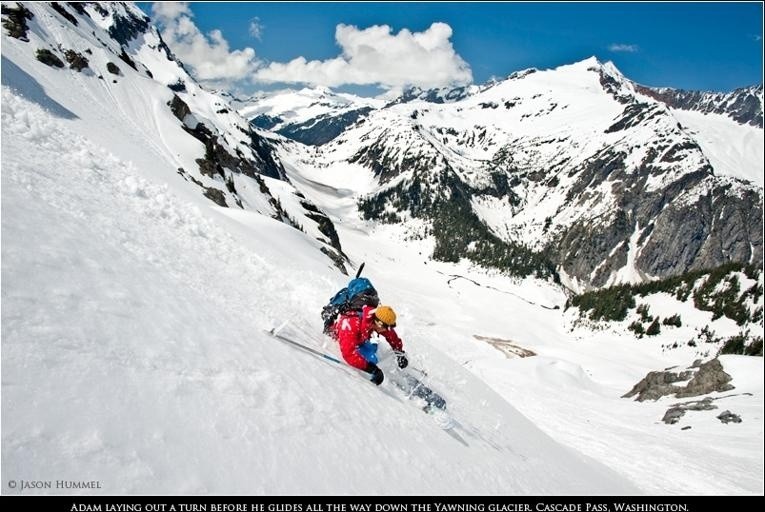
[329,305,407,385]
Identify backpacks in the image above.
[321,278,379,331]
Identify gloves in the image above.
[366,361,383,385]
[397,354,407,369]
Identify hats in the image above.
[375,306,396,325]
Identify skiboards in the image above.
[374,358,454,430]
